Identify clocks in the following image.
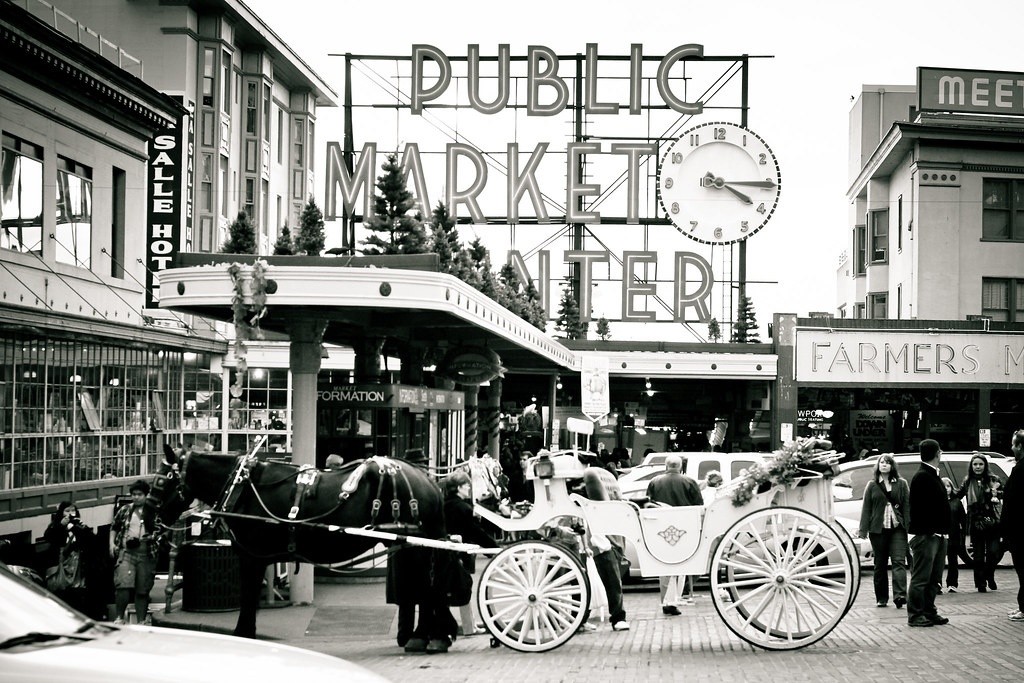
[655,121,782,245]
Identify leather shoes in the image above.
[908,612,933,628]
[929,613,950,625]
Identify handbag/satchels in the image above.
[972,515,996,534]
[45,551,88,590]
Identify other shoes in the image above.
[1007,608,1024,621]
[663,605,682,616]
[585,622,597,631]
[986,573,997,590]
[895,596,908,608]
[978,586,986,593]
[878,597,888,606]
[138,619,149,624]
[465,626,486,636]
[614,620,629,631]
[114,615,126,623]
[947,586,958,593]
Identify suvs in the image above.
[830,450,1016,566]
[619,448,776,492]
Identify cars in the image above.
[0,562,393,683]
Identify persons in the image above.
[955,452,1005,593]
[510,451,535,504]
[938,476,966,594]
[998,429,1024,622]
[109,480,163,626]
[646,454,704,616]
[907,439,952,627]
[858,452,910,609]
[382,446,477,647]
[590,447,630,468]
[44,500,94,613]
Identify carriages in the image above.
[145,440,863,655]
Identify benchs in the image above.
[522,449,622,507]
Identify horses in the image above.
[143,443,450,653]
